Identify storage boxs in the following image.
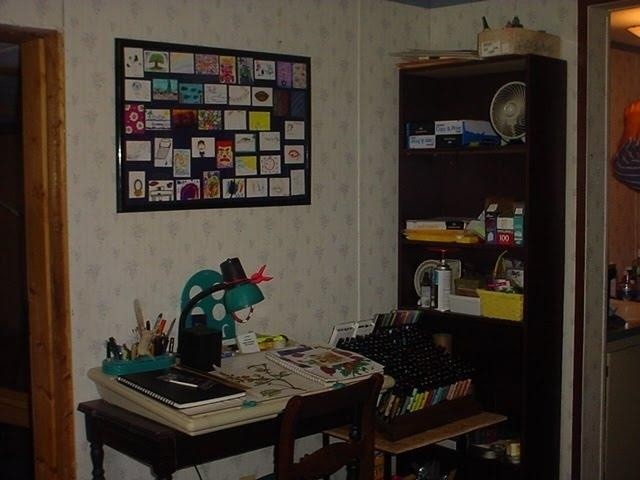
[476,28,562,59]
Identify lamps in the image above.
[176,257,266,358]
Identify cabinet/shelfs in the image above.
[398,54,567,480]
[603,333,640,480]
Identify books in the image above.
[264,343,385,383]
[118,366,247,409]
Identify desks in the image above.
[77,399,356,480]
[321,409,507,480]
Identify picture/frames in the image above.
[114,37,312,214]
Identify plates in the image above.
[413,259,441,298]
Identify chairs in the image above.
[256,371,384,480]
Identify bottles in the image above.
[419,271,432,308]
[505,439,520,463]
[505,16,524,27]
[608,259,640,301]
[481,16,492,32]
[435,249,453,311]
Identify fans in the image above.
[489,80,526,146]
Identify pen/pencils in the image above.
[336,322,472,424]
[161,378,199,388]
[135,299,176,336]
